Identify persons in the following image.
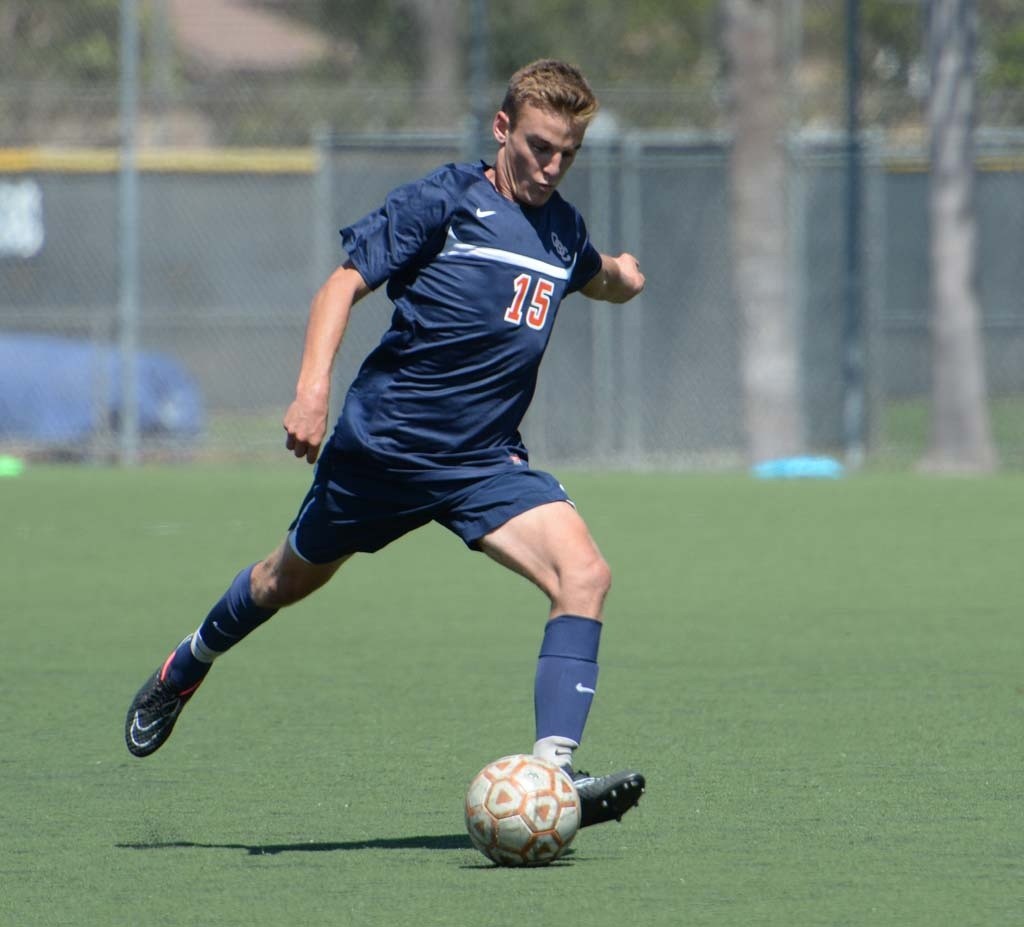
[123,59,646,833]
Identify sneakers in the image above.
[562,761,646,829]
[126,632,216,757]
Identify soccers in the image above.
[464,754,582,868]
